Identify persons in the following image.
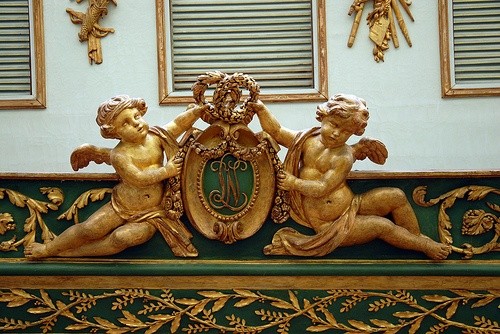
[24,95,210,257]
[240,93,452,260]
